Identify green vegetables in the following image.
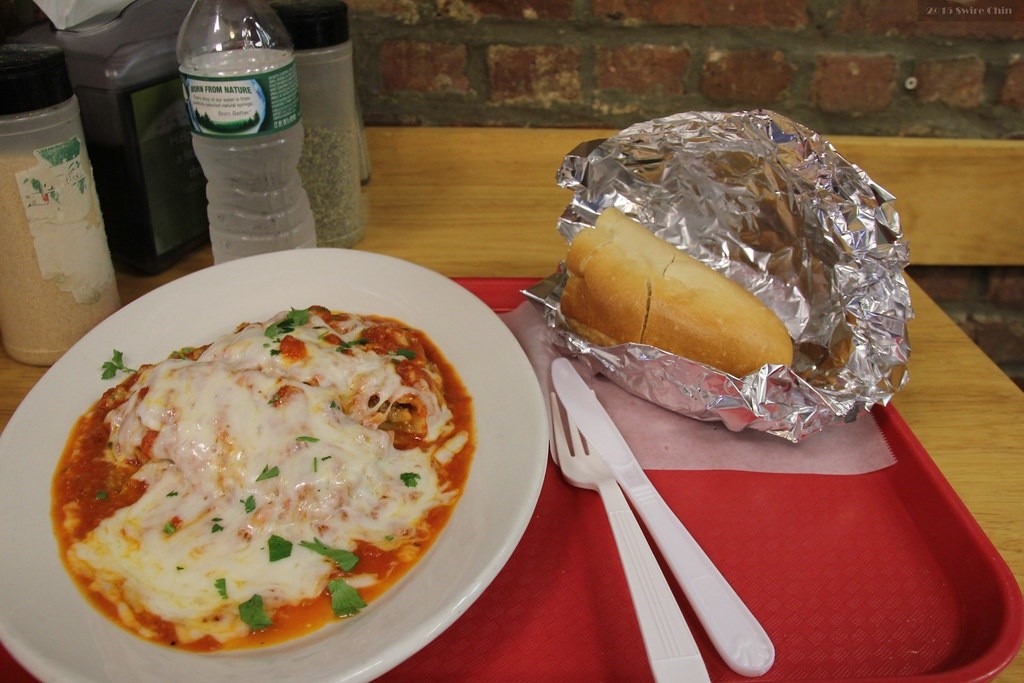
[94,307,421,631]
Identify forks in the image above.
[551,391,712,683]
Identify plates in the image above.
[0,248,547,683]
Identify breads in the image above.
[559,207,793,379]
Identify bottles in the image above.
[178,0,317,265]
[271,0,361,247]
[0,44,120,367]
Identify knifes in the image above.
[549,357,775,676]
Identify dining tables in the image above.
[0,125,1023,683]
